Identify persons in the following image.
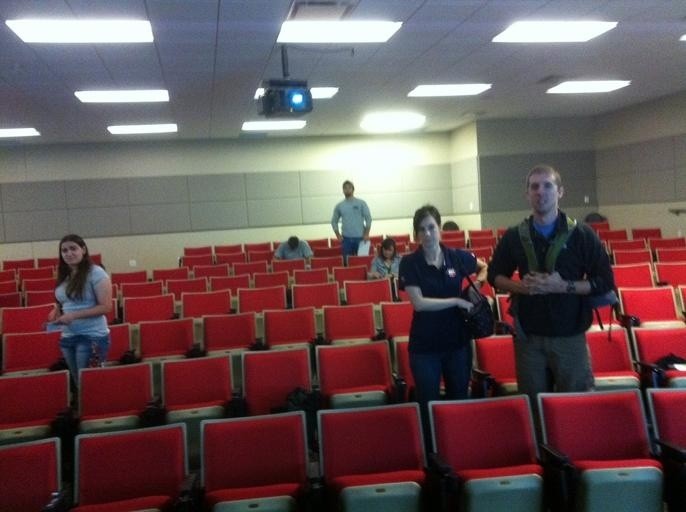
[487,164,615,393]
[273,236,313,261]
[399,204,489,403]
[367,238,403,279]
[332,180,372,257]
[43,235,113,387]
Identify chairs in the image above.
[462,274,495,297]
[153,266,189,286]
[272,260,305,270]
[347,256,374,272]
[469,236,497,247]
[409,243,418,252]
[19,266,53,279]
[648,237,686,248]
[0,269,16,280]
[293,268,328,284]
[654,261,686,286]
[510,271,521,294]
[632,229,662,239]
[215,245,242,252]
[613,249,653,265]
[394,278,410,301]
[203,311,257,357]
[105,298,117,324]
[200,411,312,512]
[194,264,228,278]
[0,293,22,308]
[497,227,507,238]
[247,250,272,262]
[123,293,176,324]
[255,272,290,288]
[618,285,686,328]
[440,231,465,238]
[496,294,514,329]
[238,287,287,312]
[311,255,344,273]
[538,388,665,512]
[1,369,71,437]
[679,284,686,316]
[273,241,282,249]
[656,248,686,262]
[308,239,329,247]
[244,243,271,251]
[322,302,376,346]
[428,394,544,512]
[184,247,212,254]
[468,230,493,237]
[315,340,396,408]
[3,259,34,270]
[121,280,164,296]
[183,289,231,317]
[4,331,62,376]
[262,307,317,350]
[381,301,414,339]
[377,241,407,256]
[210,273,249,296]
[317,402,433,512]
[182,254,211,270]
[79,363,154,433]
[111,270,147,286]
[292,281,341,307]
[38,258,61,267]
[167,277,206,301]
[588,304,622,330]
[333,264,368,289]
[111,283,118,298]
[598,230,628,243]
[234,262,267,273]
[330,239,340,247]
[440,238,464,250]
[90,255,102,266]
[469,246,494,265]
[396,336,472,402]
[647,389,686,512]
[217,253,245,264]
[314,247,340,257]
[241,347,314,416]
[591,223,610,231]
[73,422,198,512]
[470,335,518,393]
[107,324,132,367]
[1,303,57,333]
[343,278,394,306]
[25,290,57,306]
[386,235,410,242]
[585,324,641,390]
[612,262,655,289]
[140,317,195,361]
[630,320,686,388]
[369,235,383,243]
[160,354,235,422]
[0,437,62,512]
[0,280,16,293]
[609,238,647,254]
[22,278,57,291]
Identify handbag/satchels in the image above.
[450,247,497,341]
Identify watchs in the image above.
[567,280,577,296]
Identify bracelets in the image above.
[477,279,484,288]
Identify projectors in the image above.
[258,78,313,117]
